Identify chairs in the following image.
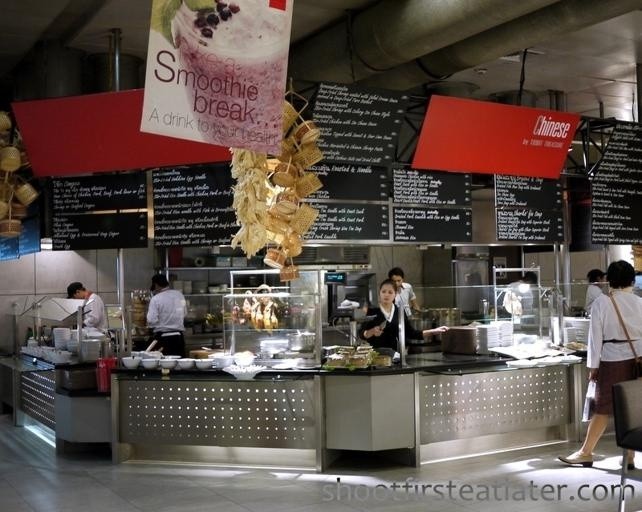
[612,379,641,512]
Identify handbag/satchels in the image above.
[634,360,642,376]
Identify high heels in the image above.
[619,461,635,470]
[558,456,592,467]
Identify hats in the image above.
[65,282,82,298]
[149,273,170,290]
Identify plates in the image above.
[193,281,209,294]
[565,343,587,351]
[258,338,288,352]
[562,318,589,343]
[80,327,109,361]
[477,320,514,348]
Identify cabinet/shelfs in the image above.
[166,265,377,336]
[221,295,323,372]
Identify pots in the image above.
[408,307,461,343]
[285,331,317,352]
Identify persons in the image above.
[359,279,449,361]
[67,282,107,358]
[387,267,420,318]
[243,290,257,312]
[557,261,642,470]
[146,274,191,359]
[502,271,537,321]
[246,285,280,340]
[584,268,608,315]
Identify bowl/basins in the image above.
[52,328,78,352]
[136,326,155,335]
[172,280,192,294]
[119,350,257,369]
[209,283,228,294]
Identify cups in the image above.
[172,10,289,152]
